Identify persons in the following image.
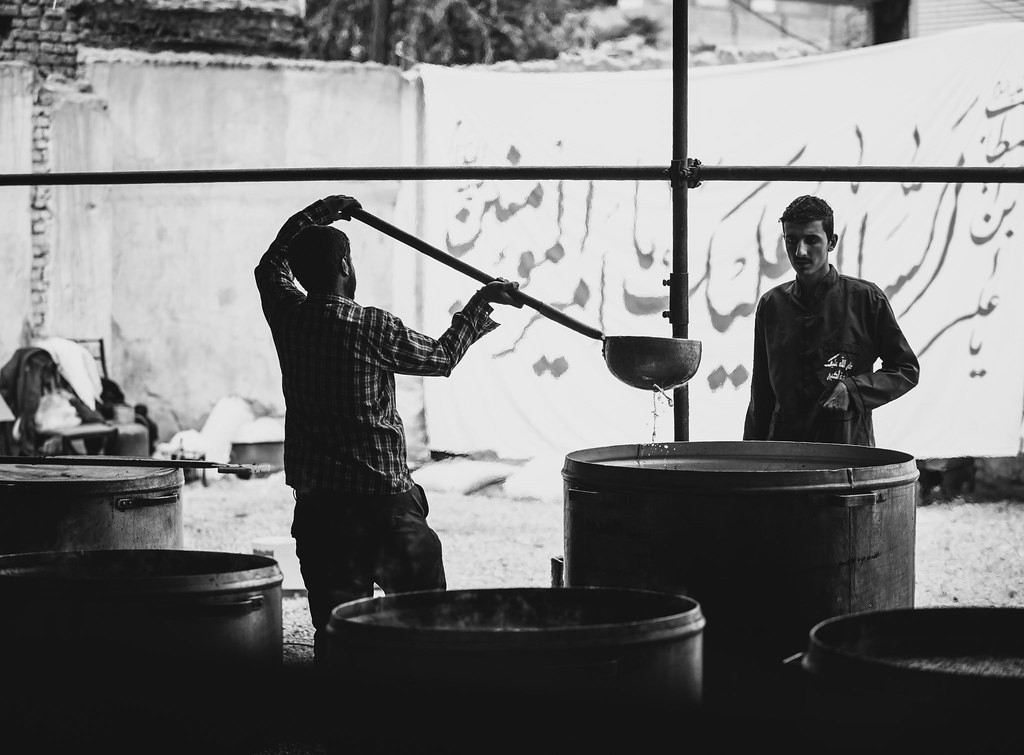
[255,194,526,658]
[743,195,919,448]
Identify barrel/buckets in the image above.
[326,587,705,755]
[560,441,919,608]
[0,454,185,550]
[1,552,284,755]
[804,607,1024,753]
